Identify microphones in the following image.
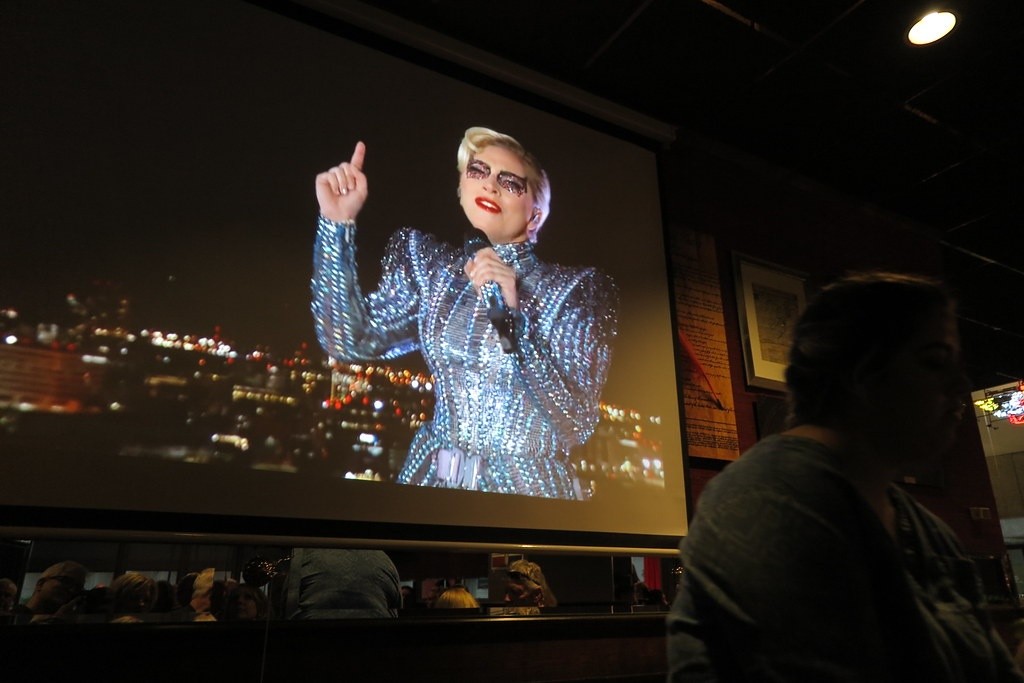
[464,228,517,353]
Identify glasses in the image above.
[50,574,81,595]
[503,570,541,585]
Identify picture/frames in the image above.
[730,249,812,397]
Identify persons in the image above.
[56,572,228,623]
[665,275,1024,683]
[307,124,621,501]
[228,583,269,620]
[282,549,399,619]
[9,561,88,624]
[503,559,557,607]
[437,588,480,608]
